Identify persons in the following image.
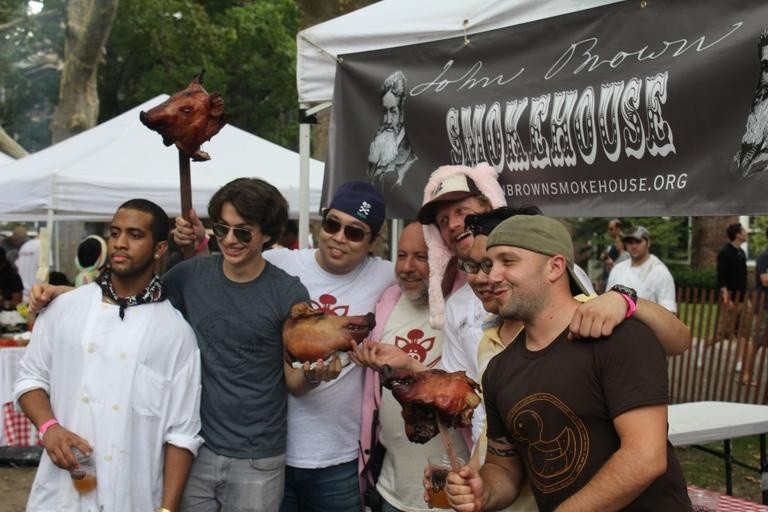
[361,71,424,219]
[734,226,767,386]
[702,223,751,373]
[734,25,767,177]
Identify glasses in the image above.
[460,259,493,276]
[211,221,262,243]
[322,216,374,242]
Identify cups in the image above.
[68,450,98,494]
[425,453,467,510]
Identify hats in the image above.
[416,173,483,226]
[619,224,653,243]
[322,180,385,236]
[485,212,592,299]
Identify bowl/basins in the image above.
[0,334,31,346]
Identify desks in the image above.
[660,399,768,507]
